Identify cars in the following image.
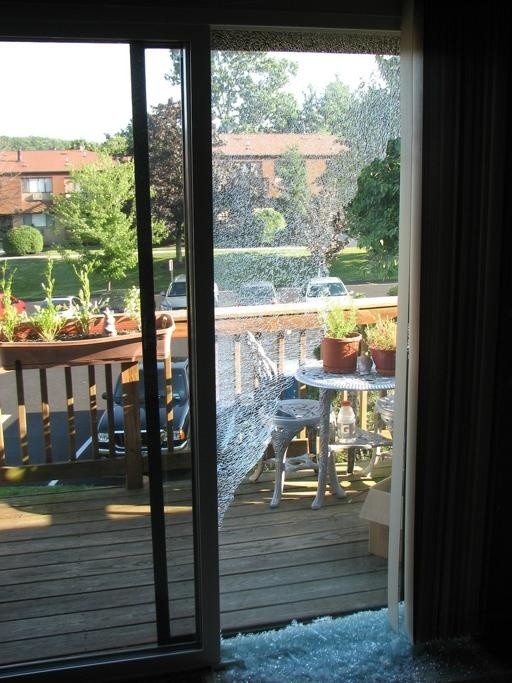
[31,295,92,319]
[298,276,354,303]
[0,293,26,322]
[159,272,219,311]
[236,282,276,307]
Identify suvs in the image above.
[97,359,189,457]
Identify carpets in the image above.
[206,601,511,683]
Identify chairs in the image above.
[245,329,348,509]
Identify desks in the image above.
[295,358,397,510]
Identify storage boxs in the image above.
[346,472,392,558]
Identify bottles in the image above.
[359,350,371,376]
[337,400,357,444]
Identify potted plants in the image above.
[1,247,177,371]
[318,291,399,379]
